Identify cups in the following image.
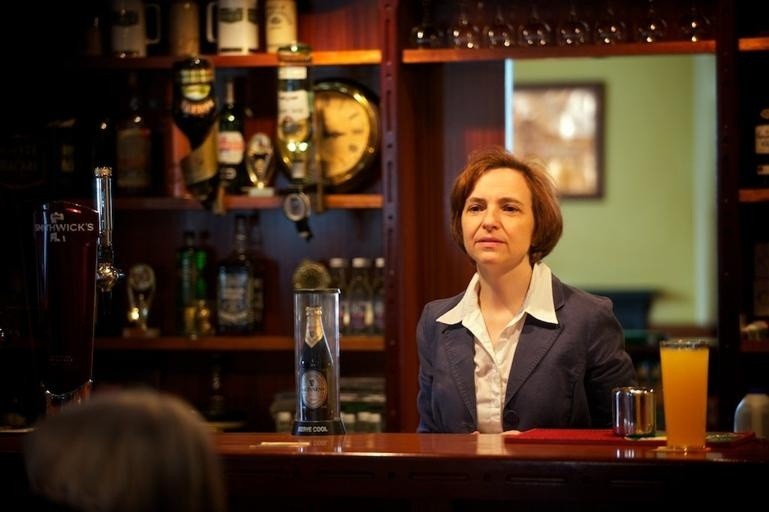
[659,340,709,448]
[611,387,656,439]
[733,393,769,439]
[206,1,261,55]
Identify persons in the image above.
[20,386,227,512]
[415,143,640,432]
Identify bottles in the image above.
[265,0,298,53]
[25,123,100,423]
[172,3,200,55]
[301,306,336,420]
[276,69,313,194]
[216,81,246,194]
[117,75,150,188]
[176,214,265,342]
[329,258,347,336]
[111,0,146,58]
[372,257,386,335]
[346,258,374,335]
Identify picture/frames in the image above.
[515,80,606,200]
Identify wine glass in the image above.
[444,1,483,49]
[637,1,672,41]
[676,0,712,40]
[594,1,628,43]
[518,1,553,48]
[484,1,517,48]
[409,3,443,47]
[556,1,592,46]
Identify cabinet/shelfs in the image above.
[0,5,417,432]
